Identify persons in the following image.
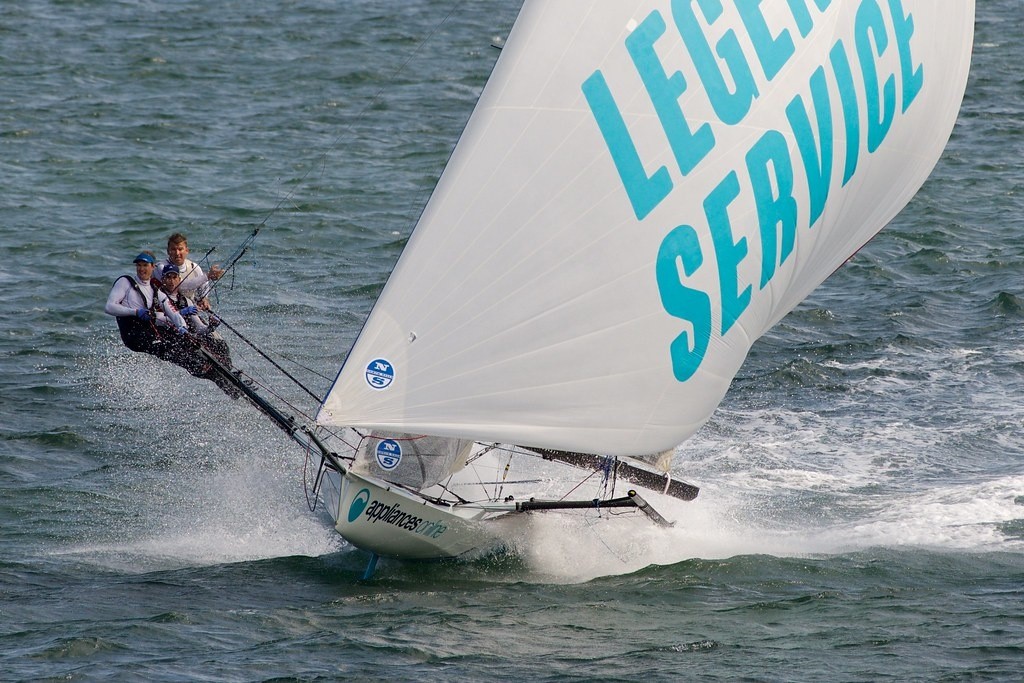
[154,234,233,369]
[105,250,211,375]
[157,265,207,335]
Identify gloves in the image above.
[180,326,188,335]
[180,306,198,315]
[136,308,152,320]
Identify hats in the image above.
[161,265,179,278]
[133,253,154,263]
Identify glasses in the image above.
[166,273,178,278]
